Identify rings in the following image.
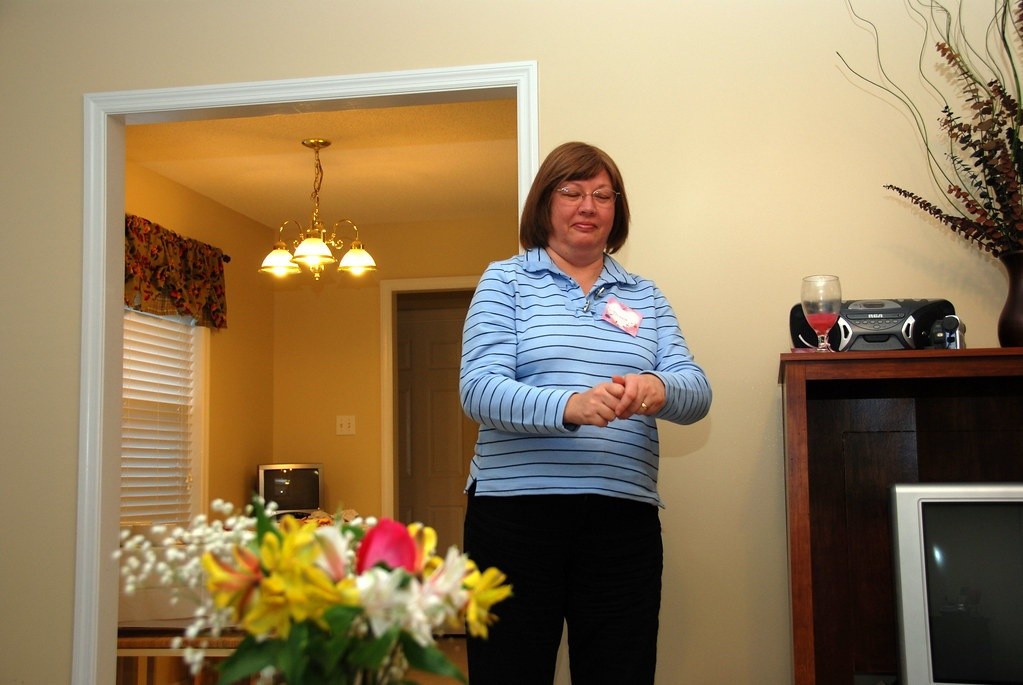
[642,402,648,410]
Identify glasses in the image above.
[553,186,621,210]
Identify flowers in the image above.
[110,496,511,685]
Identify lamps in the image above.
[256,139,380,279]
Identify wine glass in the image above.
[801,274,842,353]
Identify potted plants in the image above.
[835,0,1023,346]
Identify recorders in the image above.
[790,298,955,350]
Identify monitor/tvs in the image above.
[258,463,324,515]
[892,484,1023,685]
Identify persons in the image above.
[460,142,712,685]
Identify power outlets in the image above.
[336,415,355,435]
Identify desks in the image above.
[116,633,272,685]
